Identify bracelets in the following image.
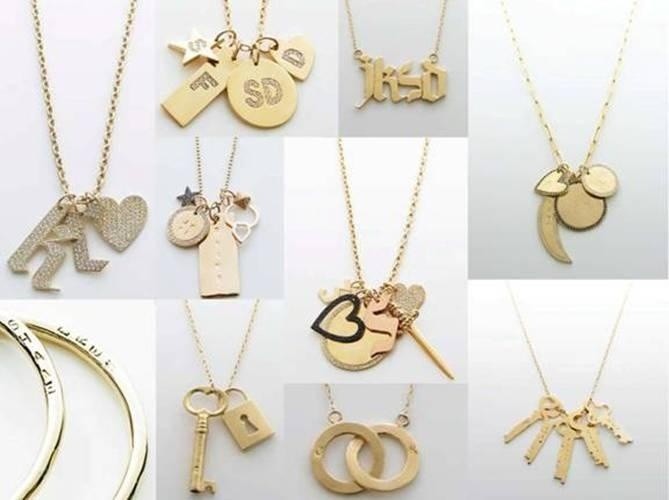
[17,318,148,500]
[0,315,66,500]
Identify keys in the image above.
[182,385,230,494]
[503,396,633,485]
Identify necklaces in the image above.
[172,301,279,496]
[3,0,151,295]
[312,384,426,496]
[157,0,319,132]
[310,138,457,381]
[500,282,635,488]
[343,1,454,114]
[493,1,641,265]
[165,135,262,298]
